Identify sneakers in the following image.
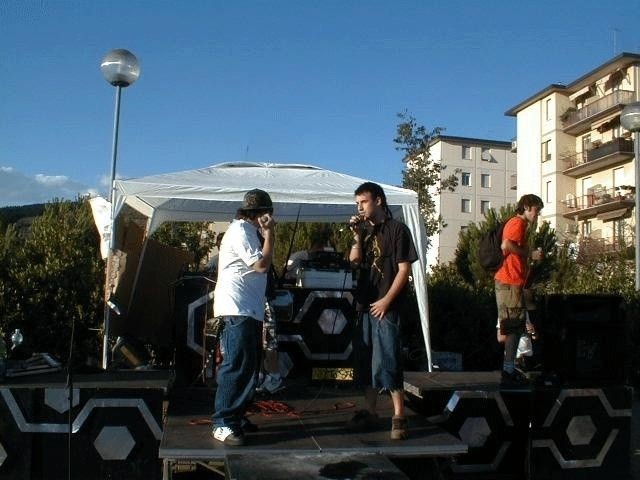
[390,416,409,440]
[501,371,529,387]
[213,426,247,446]
[239,418,257,431]
[344,409,377,432]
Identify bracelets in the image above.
[530,332,536,336]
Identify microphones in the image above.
[531,247,542,265]
[347,214,364,229]
[261,216,275,241]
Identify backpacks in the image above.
[476,216,516,272]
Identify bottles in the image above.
[10,328,24,351]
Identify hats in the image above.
[241,189,273,211]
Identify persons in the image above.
[284,233,329,271]
[479,194,544,384]
[199,232,225,273]
[348,183,419,441]
[495,310,540,368]
[210,189,275,447]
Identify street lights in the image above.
[619,100,640,293]
[100,48,142,202]
